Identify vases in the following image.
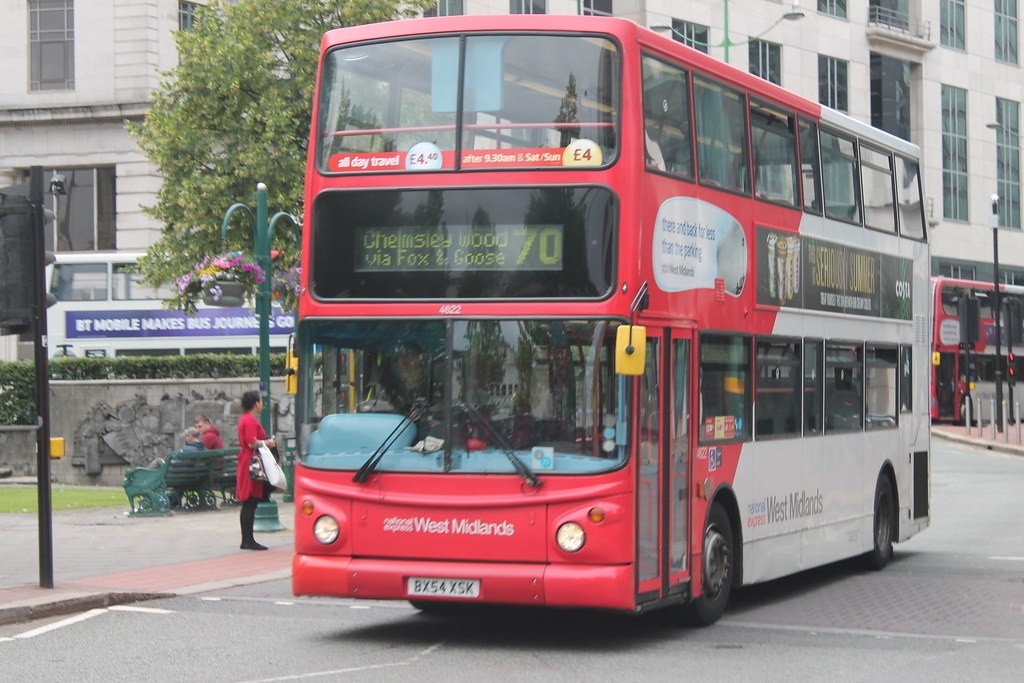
[200,279,247,306]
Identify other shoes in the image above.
[240,542,268,550]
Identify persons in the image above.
[138,428,209,510]
[160,415,223,508]
[358,340,433,445]
[237,391,276,550]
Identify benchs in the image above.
[122,446,244,516]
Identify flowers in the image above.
[269,266,307,315]
[179,246,262,314]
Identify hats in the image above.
[180,427,200,438]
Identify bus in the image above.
[284,15,933,628]
[45,251,295,358]
[931,275,1024,424]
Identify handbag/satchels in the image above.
[248,441,268,481]
[258,440,288,491]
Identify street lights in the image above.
[651,0,805,64]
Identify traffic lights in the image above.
[1008,352,1015,386]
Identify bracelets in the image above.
[268,440,271,447]
